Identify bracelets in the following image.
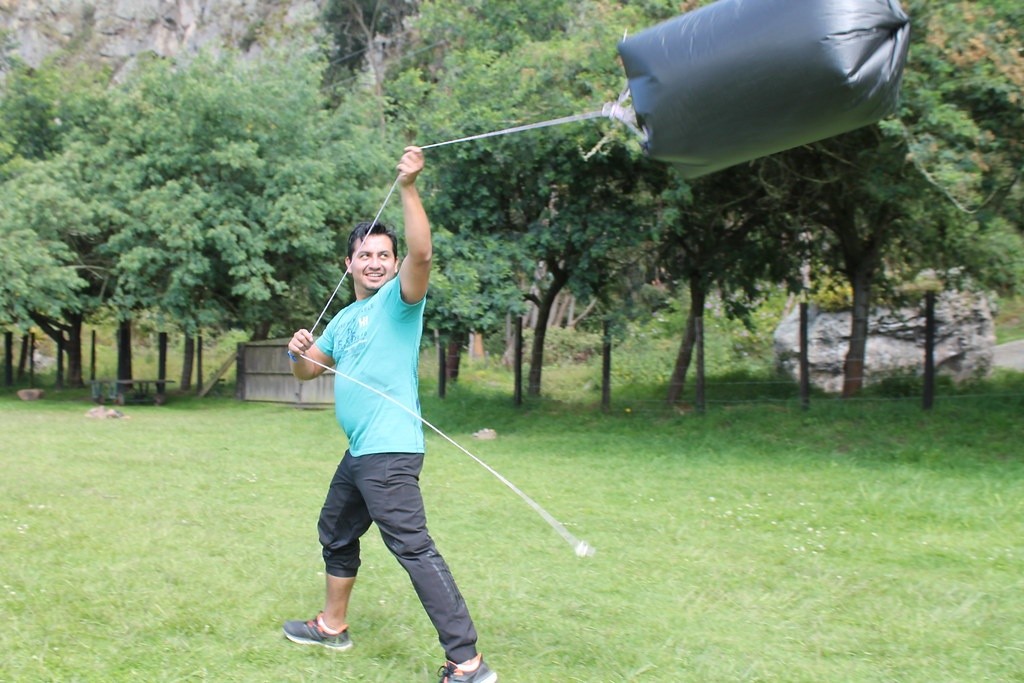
[288,350,299,362]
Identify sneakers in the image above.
[282,610,353,652]
[437,652,497,683]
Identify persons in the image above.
[282,146,500,683]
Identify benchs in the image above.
[87,377,176,405]
